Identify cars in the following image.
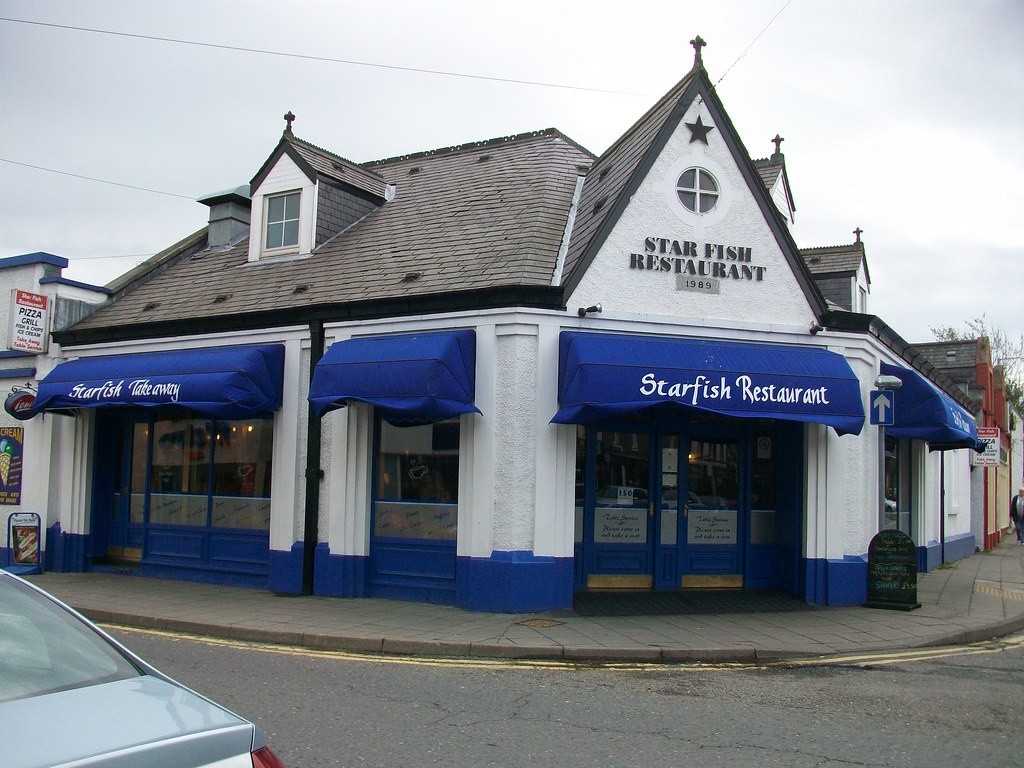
[884,499,897,512]
[0,569,291,768]
[596,485,647,509]
[700,497,727,507]
[663,491,701,511]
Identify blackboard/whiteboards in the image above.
[867,529,918,604]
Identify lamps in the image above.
[578,303,603,320]
[809,320,824,335]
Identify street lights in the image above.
[874,372,903,533]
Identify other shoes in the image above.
[1016,540,1020,545]
[1022,543,1024,546]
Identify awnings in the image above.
[547,330,867,438]
[30,341,285,419]
[307,329,484,428]
[879,360,985,453]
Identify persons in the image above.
[1009,486,1024,544]
